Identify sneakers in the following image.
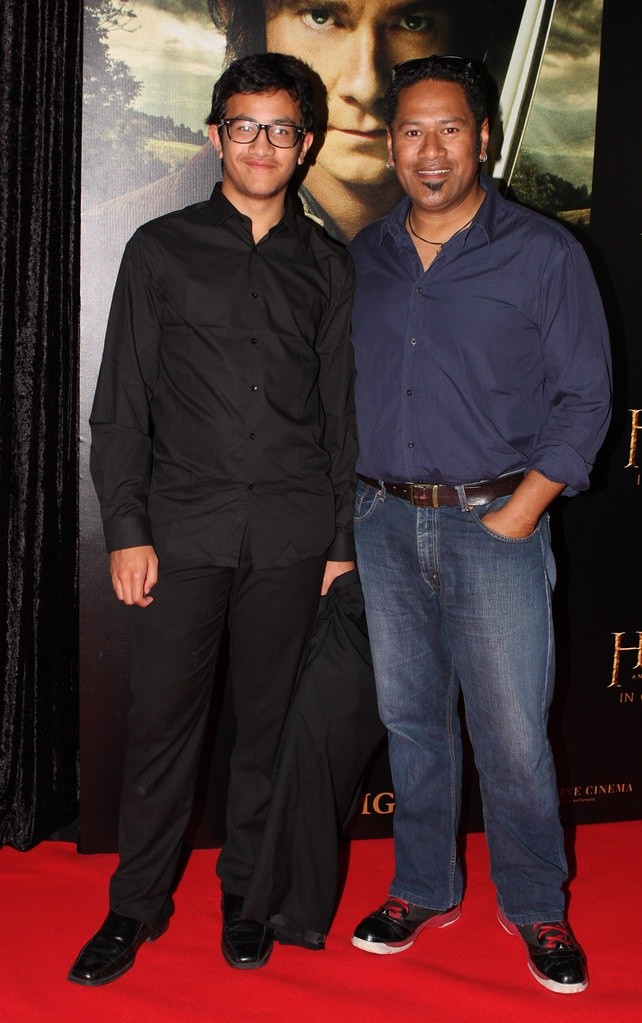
[351,893,587,994]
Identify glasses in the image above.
[220,117,306,149]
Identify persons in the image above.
[66,54,357,985]
[342,54,612,998]
[79,0,509,434]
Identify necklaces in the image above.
[408,204,476,248]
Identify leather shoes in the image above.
[69,893,273,988]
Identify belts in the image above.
[358,466,523,507]
